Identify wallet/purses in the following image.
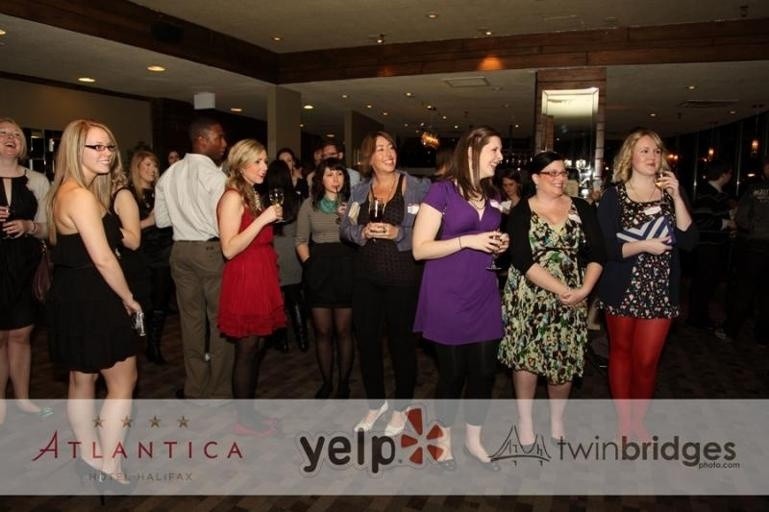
[348,201,361,228]
[131,312,146,337]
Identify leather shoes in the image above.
[314,382,655,470]
[94,470,136,503]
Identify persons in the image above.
[0,114,768,501]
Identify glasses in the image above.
[84,145,118,152]
[540,170,569,177]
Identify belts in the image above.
[177,237,220,243]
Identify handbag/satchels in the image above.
[616,215,676,247]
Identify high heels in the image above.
[76,457,101,495]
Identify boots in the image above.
[277,332,289,353]
[290,304,309,350]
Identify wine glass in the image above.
[268,187,287,224]
[485,231,505,272]
[1,206,18,242]
[652,166,670,207]
[336,192,348,224]
[368,197,385,244]
